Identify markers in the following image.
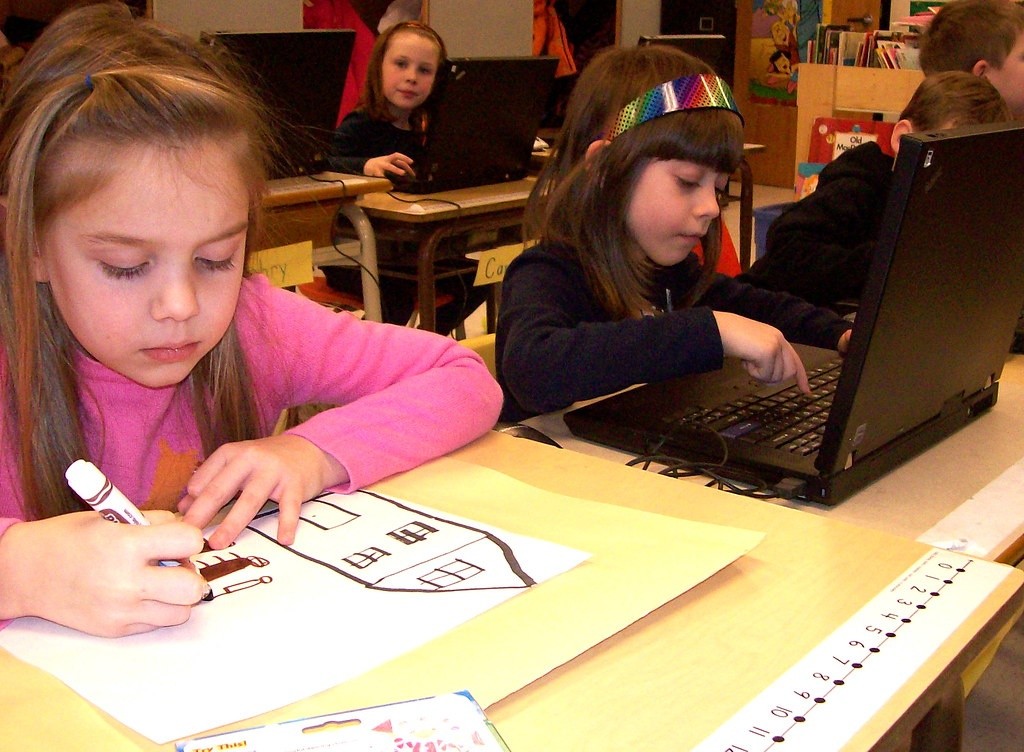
[64,459,213,603]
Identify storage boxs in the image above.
[835,63,926,113]
[728,63,833,189]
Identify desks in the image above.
[0,145,1024,752]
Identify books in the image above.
[803,6,943,69]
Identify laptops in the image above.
[562,120,1024,506]
[196,27,357,178]
[380,55,564,195]
[637,34,728,83]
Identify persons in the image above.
[0,3,503,639]
[488,45,859,429]
[733,0,1024,306]
[308,20,491,336]
